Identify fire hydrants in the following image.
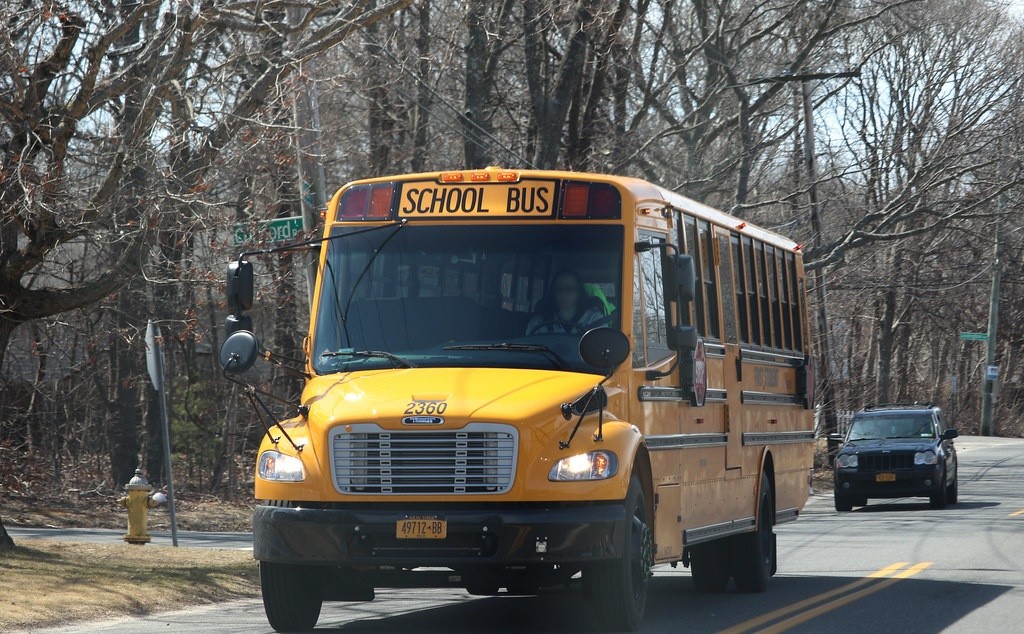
[115,469,161,544]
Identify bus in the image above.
[221,165,822,634]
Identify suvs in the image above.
[829,401,959,513]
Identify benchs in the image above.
[344,296,531,355]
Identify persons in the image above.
[524,270,610,338]
